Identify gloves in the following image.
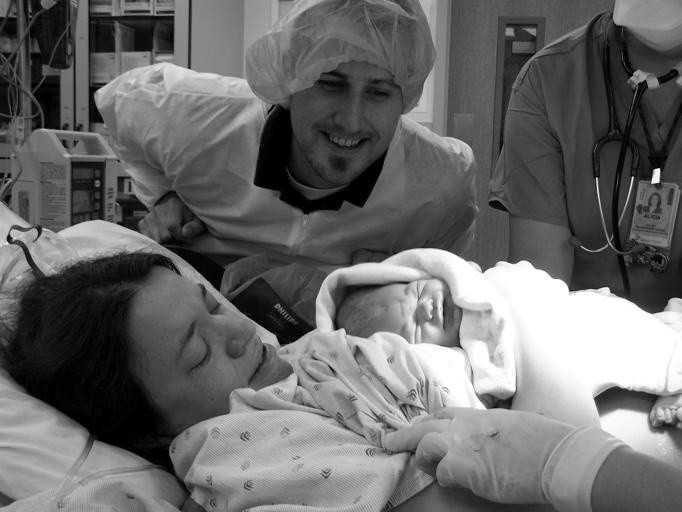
[383,406,632,511]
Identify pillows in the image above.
[1,219,278,511]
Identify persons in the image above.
[330,270,681,431]
[643,192,662,214]
[485,0,680,314]
[93,1,479,268]
[378,398,680,511]
[4,252,680,512]
